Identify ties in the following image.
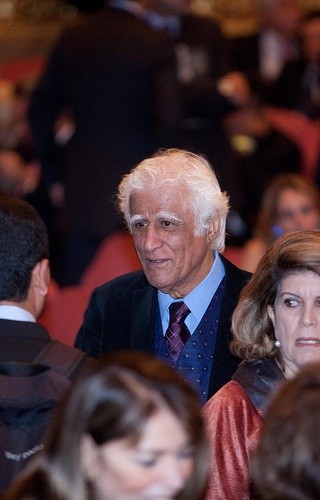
[165,301,192,367]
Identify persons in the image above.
[0,0,320,279]
[74,150,255,401]
[258,174,320,241]
[200,229,320,500]
[249,362,320,500]
[0,195,108,500]
[0,352,209,500]
